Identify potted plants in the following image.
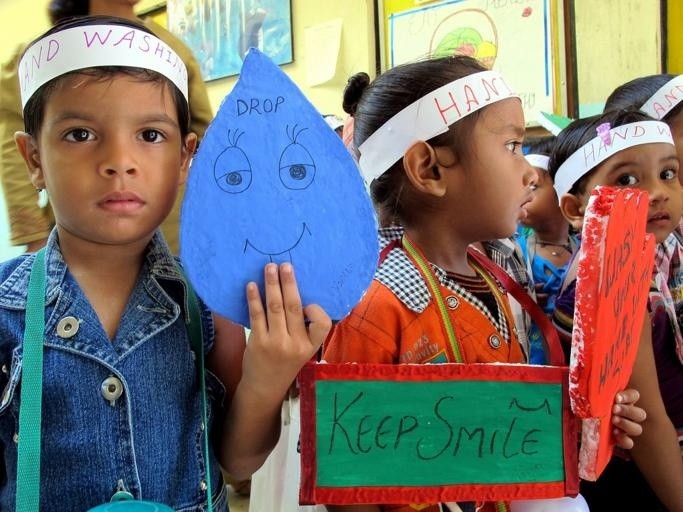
[374,1,578,153]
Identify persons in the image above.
[550,106,682,512]
[603,73,681,173]
[1,1,213,259]
[0,15,336,511]
[518,132,581,271]
[321,53,649,512]
